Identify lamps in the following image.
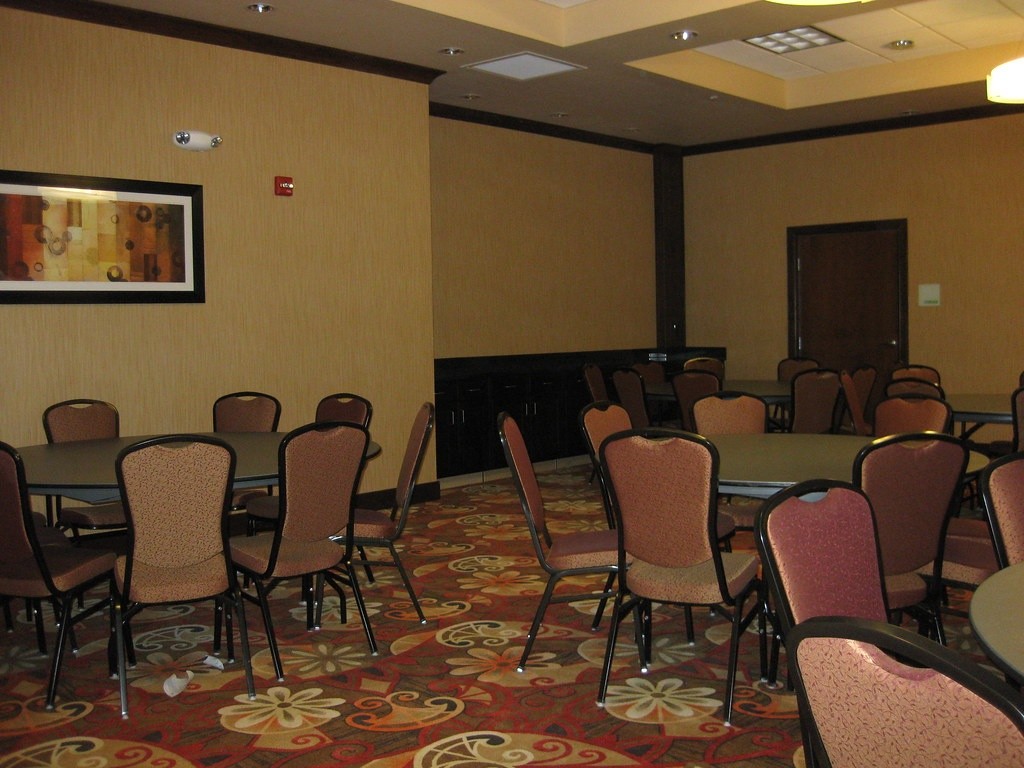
[986,57,1024,107]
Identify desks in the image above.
[966,564,1024,693]
[626,378,846,433]
[933,390,1020,440]
[702,435,993,515]
[16,430,401,532]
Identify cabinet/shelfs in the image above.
[434,346,727,479]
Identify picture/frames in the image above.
[0,170,207,306]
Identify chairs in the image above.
[0,356,1024,768]
